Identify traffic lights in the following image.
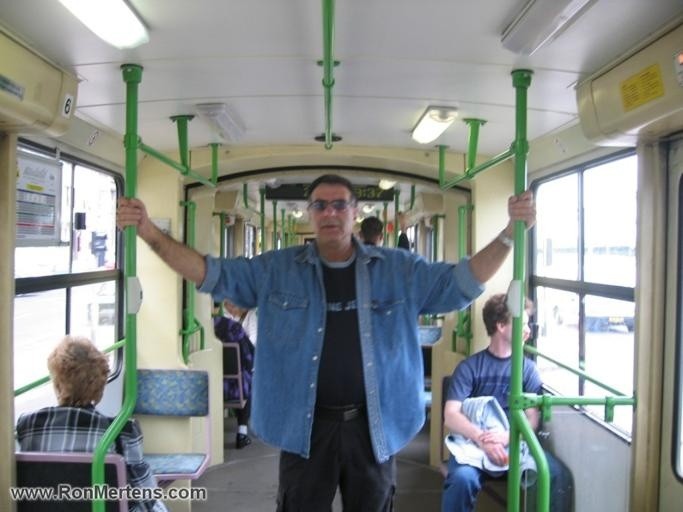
[91,233,107,254]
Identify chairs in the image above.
[14,341,248,511]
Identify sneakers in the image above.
[236,433,250,448]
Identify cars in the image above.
[549,240,634,334]
[85,281,117,326]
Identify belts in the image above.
[313,405,365,423]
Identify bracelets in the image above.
[499,229,514,248]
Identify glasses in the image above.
[307,200,352,212]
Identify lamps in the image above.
[57,0,148,50]
[195,101,247,144]
[501,1,595,58]
[411,105,458,145]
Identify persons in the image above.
[15,334,169,512]
[358,215,385,248]
[440,293,575,512]
[115,175,536,512]
[212,298,257,447]
